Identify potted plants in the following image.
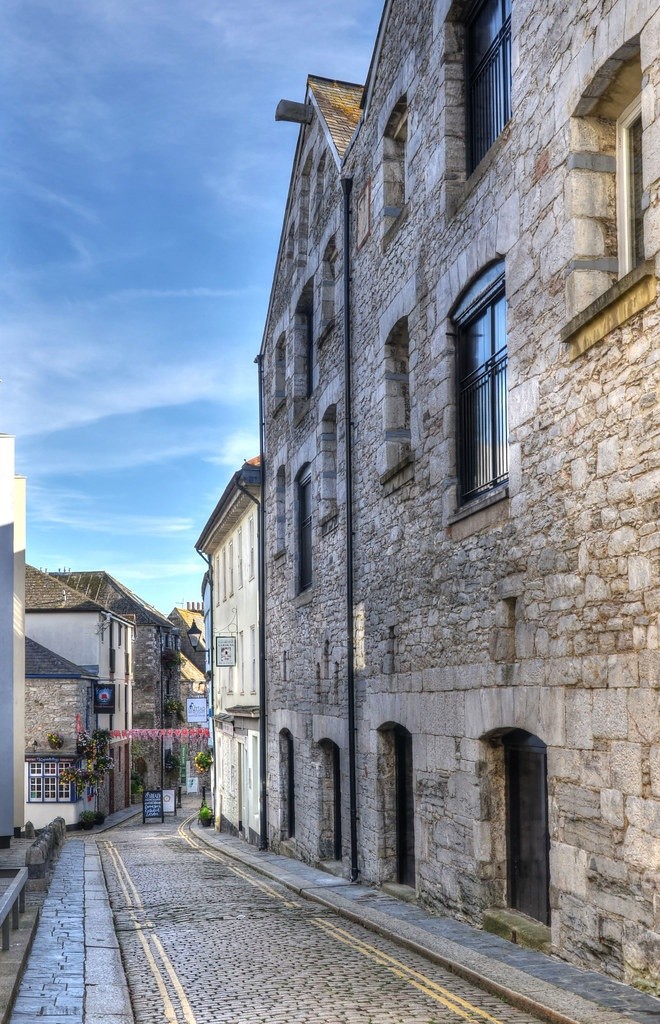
[198,804,213,827]
[95,811,106,826]
[79,810,96,830]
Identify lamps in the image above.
[186,619,202,652]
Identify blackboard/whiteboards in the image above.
[162,789,176,813]
[142,790,164,818]
[187,777,198,792]
